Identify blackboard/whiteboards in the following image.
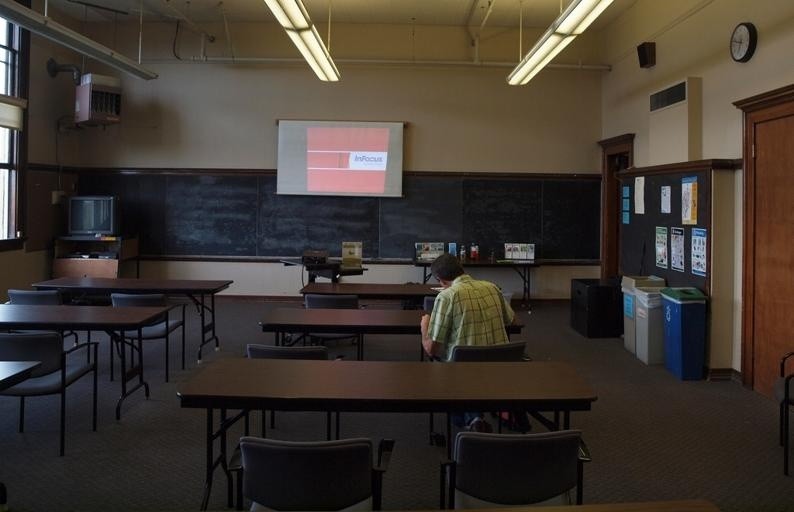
[620,168,710,291]
[68,166,604,264]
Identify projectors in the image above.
[302,250,328,264]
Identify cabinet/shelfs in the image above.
[613,157,744,384]
[50,233,142,282]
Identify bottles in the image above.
[470,242,481,259]
[488,247,495,262]
[459,244,466,260]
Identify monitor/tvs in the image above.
[68,196,114,237]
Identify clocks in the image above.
[728,20,758,61]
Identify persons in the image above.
[420,254,514,432]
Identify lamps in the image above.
[505,1,613,90]
[261,0,340,83]
[0,1,157,83]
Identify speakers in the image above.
[637,42,655,68]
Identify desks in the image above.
[478,496,719,510]
[177,283,598,511]
[1,276,234,510]
[413,258,536,312]
[281,255,365,285]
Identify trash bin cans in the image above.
[620,276,708,380]
